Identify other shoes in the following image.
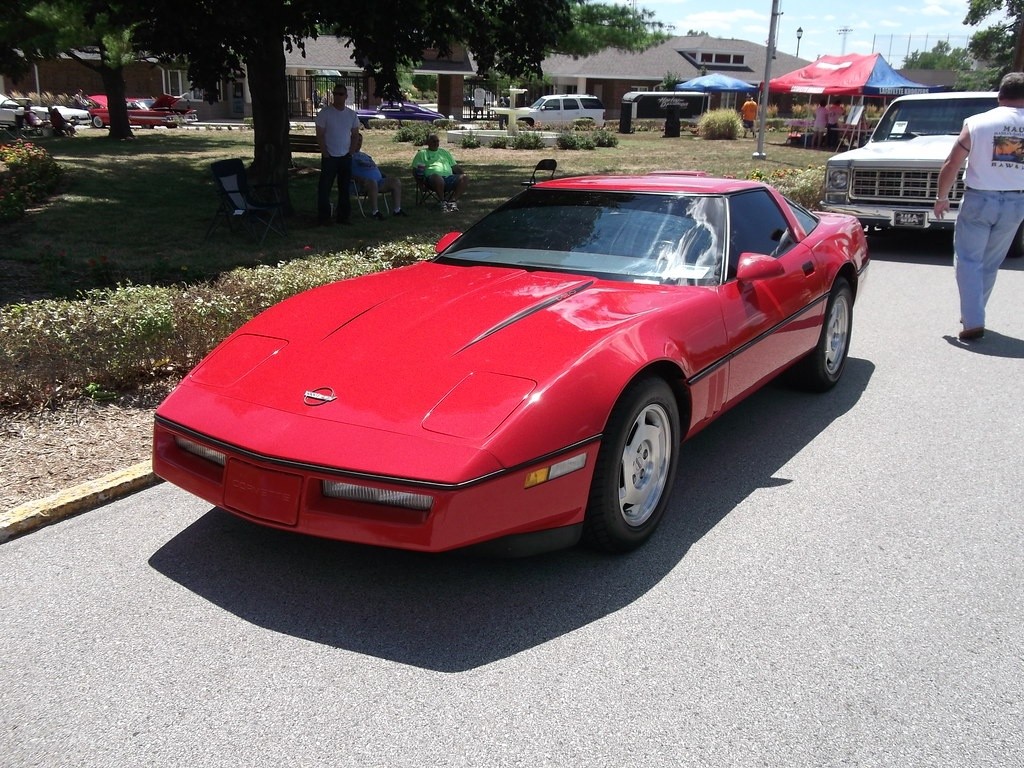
[959,326,984,338]
[448,202,460,212]
[319,219,331,225]
[439,201,449,212]
[335,216,350,224]
[394,209,408,216]
[371,211,384,220]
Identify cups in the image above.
[417,164,425,175]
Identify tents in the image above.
[758,53,945,149]
[676,73,761,114]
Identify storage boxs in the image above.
[787,134,813,147]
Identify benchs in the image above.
[288,134,323,154]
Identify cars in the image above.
[0,93,93,141]
[83,92,199,130]
[354,100,446,130]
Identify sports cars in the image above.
[152,162,874,564]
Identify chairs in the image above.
[337,173,391,218]
[521,159,557,188]
[0,110,71,141]
[536,200,715,259]
[205,158,290,244]
[412,151,458,209]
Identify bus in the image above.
[622,90,720,126]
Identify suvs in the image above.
[817,91,1024,261]
[515,93,609,132]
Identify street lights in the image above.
[795,26,804,58]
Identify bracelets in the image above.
[936,195,948,201]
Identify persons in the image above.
[74,89,90,109]
[811,101,865,148]
[315,83,359,225]
[23,104,75,136]
[412,135,468,212]
[935,72,1024,339]
[499,96,510,129]
[741,95,757,137]
[350,133,408,219]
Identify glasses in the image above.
[333,91,346,96]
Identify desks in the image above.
[785,123,873,148]
